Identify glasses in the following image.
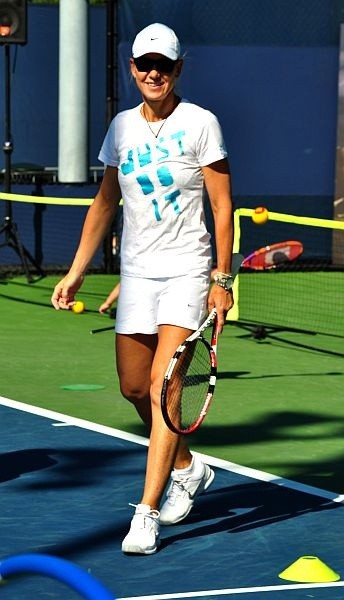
[134,56,177,74]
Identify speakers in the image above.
[0,0,27,46]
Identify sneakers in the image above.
[121,502,161,555]
[159,456,215,526]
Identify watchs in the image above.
[214,272,235,290]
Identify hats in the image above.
[132,22,181,62]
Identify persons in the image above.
[98,282,121,314]
[50,23,233,556]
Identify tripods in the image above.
[0,46,46,283]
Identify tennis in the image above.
[252,207,268,224]
[72,301,84,312]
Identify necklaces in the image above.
[143,103,175,138]
